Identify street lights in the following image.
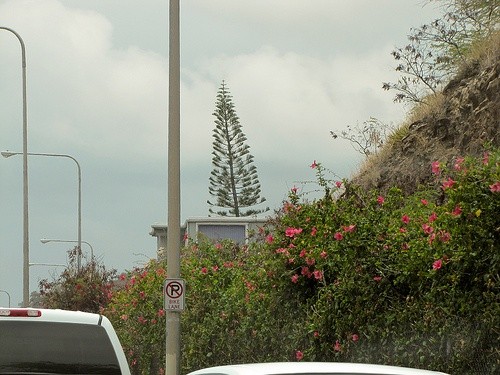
[29,263,71,270]
[2,150,82,274]
[39,238,94,266]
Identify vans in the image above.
[0,307,131,375]
